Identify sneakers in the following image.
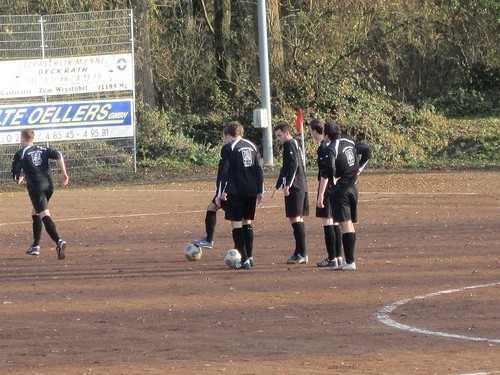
[316,258,338,267]
[333,262,357,271]
[26,246,40,255]
[56,239,67,259]
[287,253,308,264]
[192,239,213,249]
[248,257,253,266]
[236,259,251,269]
[337,257,343,266]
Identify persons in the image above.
[214,121,265,269]
[270,122,310,264]
[11,129,69,259]
[317,123,372,270]
[310,118,343,267]
[192,121,244,250]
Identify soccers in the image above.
[184,243,202,261]
[224,249,241,268]
